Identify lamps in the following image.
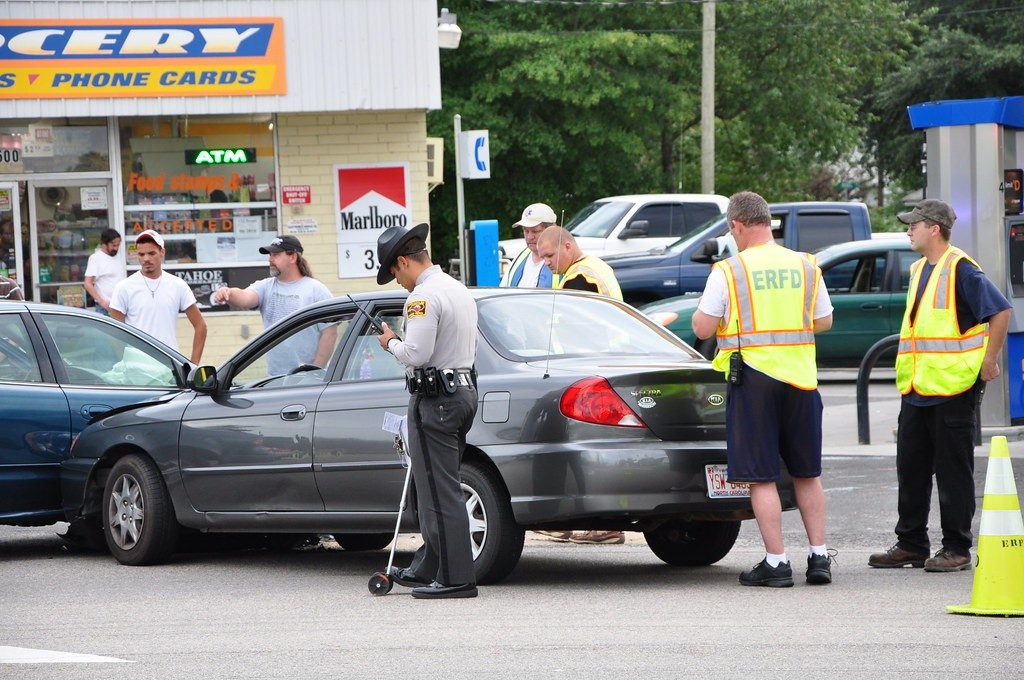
[437,7,463,49]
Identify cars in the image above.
[0,300,244,526]
[639,239,923,368]
[61,288,800,587]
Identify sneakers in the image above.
[806,553,833,583]
[738,558,794,587]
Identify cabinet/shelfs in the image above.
[39,225,105,257]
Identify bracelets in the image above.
[387,337,397,349]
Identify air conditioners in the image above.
[427,136,444,182]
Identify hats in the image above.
[259,235,303,255]
[375,222,428,284]
[512,203,557,227]
[896,200,956,232]
[135,229,165,250]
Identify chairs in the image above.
[849,258,876,293]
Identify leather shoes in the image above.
[924,547,974,574]
[411,580,478,598]
[869,545,930,570]
[385,566,431,588]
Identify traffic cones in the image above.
[944,436,1024,616]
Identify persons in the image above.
[107,230,207,367]
[499,203,565,541]
[214,235,343,541]
[532,227,630,544]
[85,228,127,372]
[377,223,480,598]
[868,198,1012,573]
[0,275,26,363]
[691,191,834,588]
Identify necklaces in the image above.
[141,270,162,298]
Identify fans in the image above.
[40,187,68,208]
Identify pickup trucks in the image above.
[603,201,889,305]
[498,194,910,284]
